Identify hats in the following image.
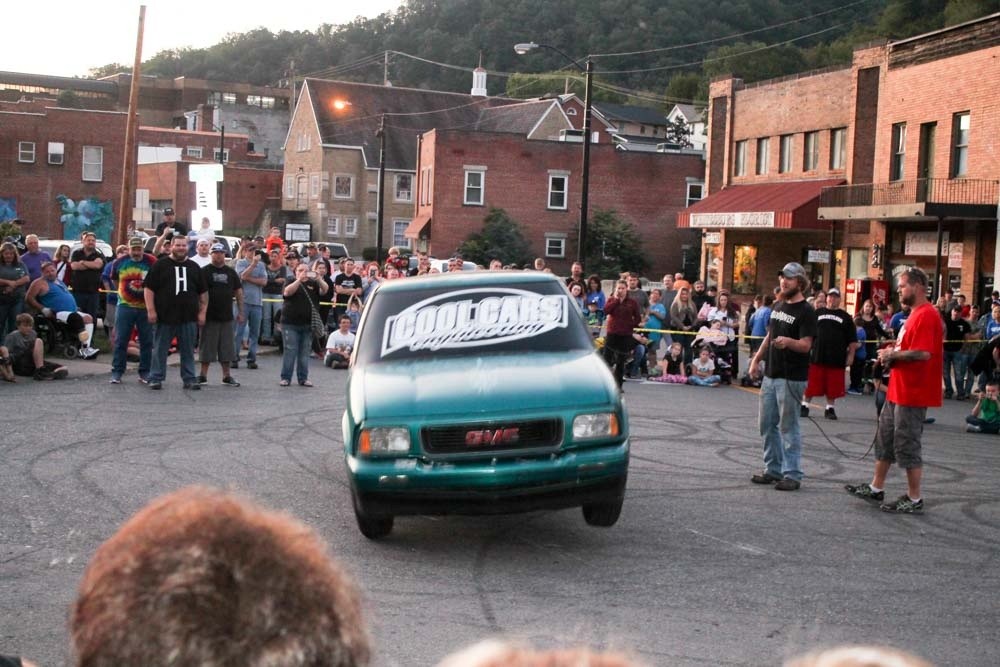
[826,287,841,297]
[163,208,174,215]
[129,237,143,247]
[210,243,227,254]
[286,252,302,259]
[778,262,806,278]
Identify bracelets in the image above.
[82,260,86,264]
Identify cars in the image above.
[289,241,352,272]
[407,255,480,272]
[326,266,634,540]
[182,235,241,261]
[35,238,116,263]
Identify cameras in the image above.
[170,228,175,233]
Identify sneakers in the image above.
[880,495,924,512]
[774,477,799,489]
[801,405,809,417]
[825,408,837,420]
[845,484,883,503]
[750,473,780,483]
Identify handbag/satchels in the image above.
[311,308,325,337]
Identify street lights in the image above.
[331,96,387,267]
[183,110,226,237]
[510,39,595,275]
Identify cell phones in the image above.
[371,270,375,276]
[723,317,726,320]
[255,249,260,255]
[306,271,317,278]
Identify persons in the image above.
[0,219,107,381]
[103,206,382,390]
[0,487,369,667]
[562,261,740,392]
[379,248,552,284]
[748,262,816,490]
[782,645,934,667]
[846,266,943,512]
[740,287,1000,433]
[437,639,639,667]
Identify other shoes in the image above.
[943,388,971,401]
[300,380,313,386]
[82,348,100,359]
[31,361,70,381]
[331,360,342,369]
[923,418,936,423]
[847,389,864,396]
[248,362,257,369]
[280,379,288,386]
[966,423,980,432]
[231,361,238,368]
[111,373,240,391]
